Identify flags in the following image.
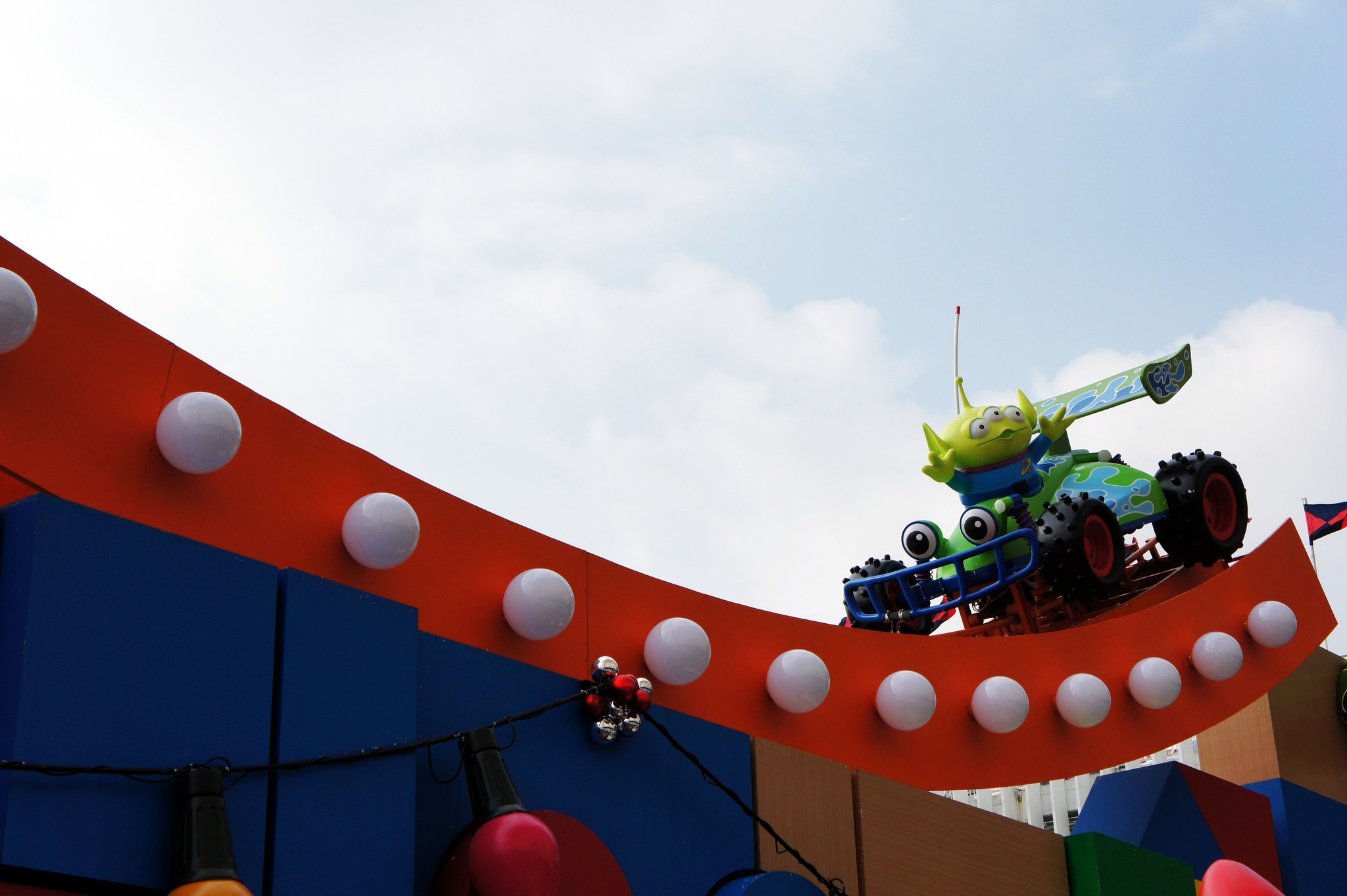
[1304,501,1347,543]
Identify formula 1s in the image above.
[842,344,1249,636]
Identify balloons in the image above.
[583,656,652,746]
